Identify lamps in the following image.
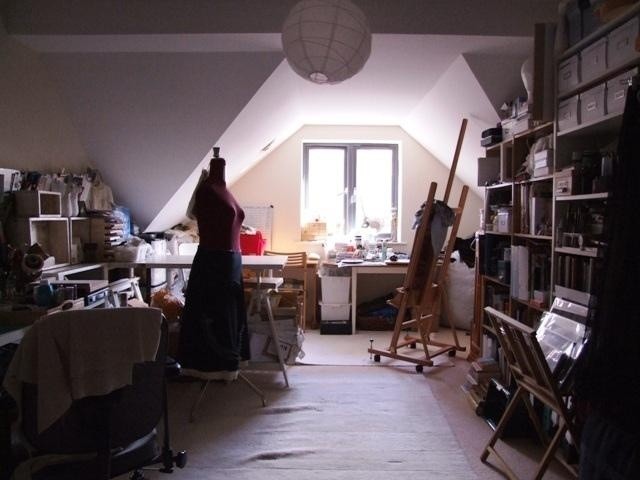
[279,1,373,87]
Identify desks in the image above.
[107,255,290,390]
[0,276,141,347]
[335,258,411,335]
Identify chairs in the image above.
[263,249,308,333]
[1,305,191,479]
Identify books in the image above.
[341,258,363,265]
[464,148,605,446]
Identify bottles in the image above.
[39,280,54,305]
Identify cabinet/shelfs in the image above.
[472,121,554,363]
[9,189,91,270]
[551,1,638,316]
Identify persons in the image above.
[178,156,252,374]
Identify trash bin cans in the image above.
[411,282,442,332]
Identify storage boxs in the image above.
[317,273,353,322]
